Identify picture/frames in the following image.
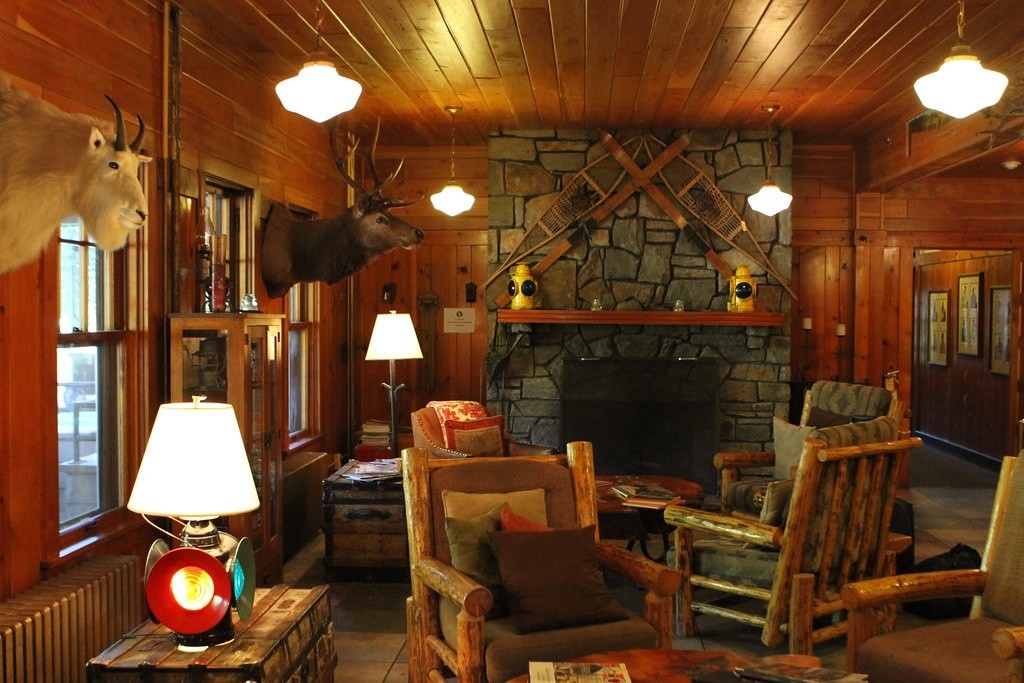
[924,288,952,369]
[954,271,985,360]
[986,284,1012,377]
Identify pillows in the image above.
[484,524,632,634]
[442,500,519,623]
[499,500,555,532]
[439,487,550,527]
[441,414,508,458]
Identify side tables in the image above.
[318,458,409,574]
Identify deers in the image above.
[262,104,425,299]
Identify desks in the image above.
[80,582,342,683]
[592,472,706,562]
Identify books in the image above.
[529,661,633,683]
[595,477,685,509]
[342,419,403,484]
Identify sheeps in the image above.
[0,72,154,274]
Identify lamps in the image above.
[273,0,364,127]
[910,0,1010,122]
[428,104,476,219]
[125,394,264,653]
[745,103,795,221]
[361,309,426,457]
[999,155,1023,173]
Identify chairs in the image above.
[711,378,908,518]
[398,440,688,683]
[660,434,913,658]
[409,398,563,459]
[840,450,1024,683]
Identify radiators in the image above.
[281,449,331,559]
[0,553,145,683]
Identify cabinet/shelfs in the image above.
[161,307,290,588]
